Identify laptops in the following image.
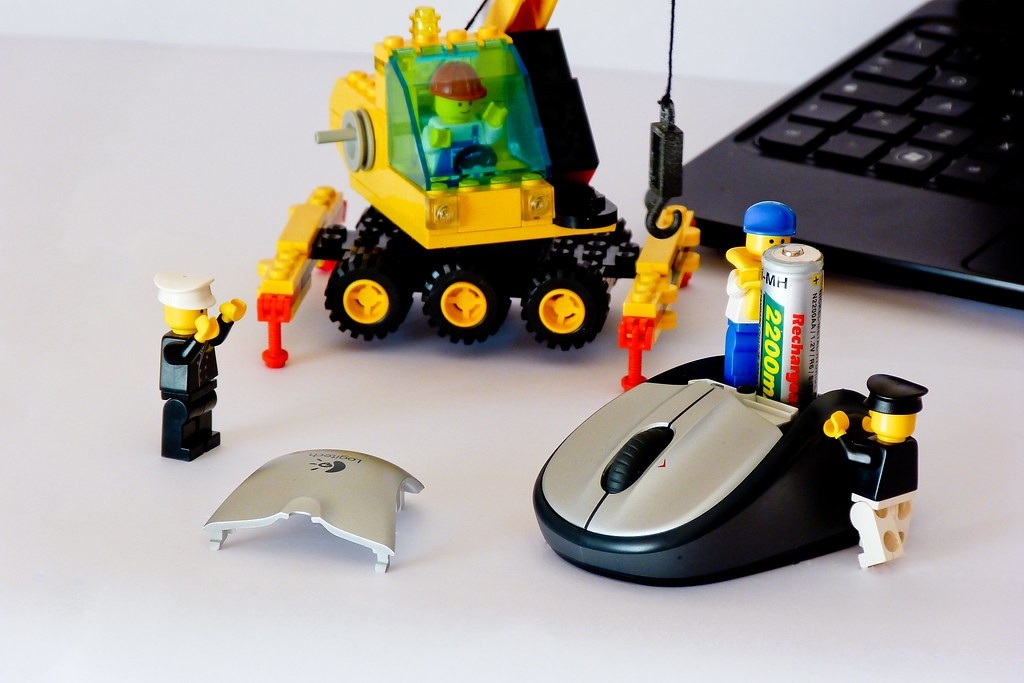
[646,0,1024,312]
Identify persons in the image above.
[823,374,929,567]
[154,271,246,462]
[724,201,796,385]
[421,61,507,187]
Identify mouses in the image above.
[533,352,876,589]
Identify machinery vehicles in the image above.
[256,0,701,393]
